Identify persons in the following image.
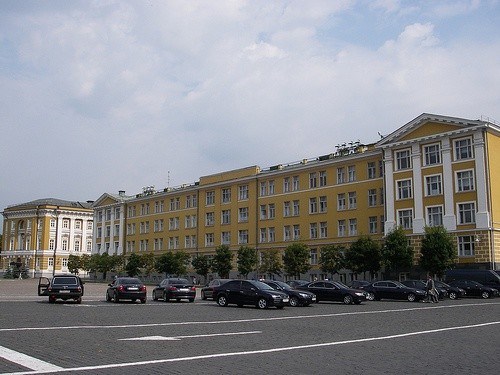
[18,273,22,280]
[423,275,439,303]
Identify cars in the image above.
[38,275,84,304]
[200,278,317,310]
[106,276,148,304]
[448,279,500,299]
[294,279,368,305]
[153,278,197,303]
[350,279,467,302]
